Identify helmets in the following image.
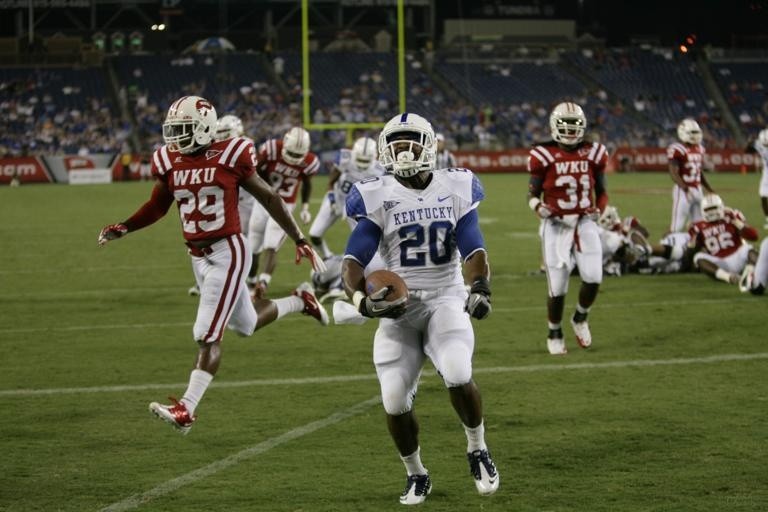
[676,117,702,146]
[350,137,377,172]
[213,114,244,143]
[280,126,310,166]
[596,205,620,230]
[376,112,437,178]
[161,94,217,156]
[547,101,586,146]
[699,193,724,224]
[758,129,768,148]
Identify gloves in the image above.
[722,206,737,224]
[299,209,311,226]
[533,201,564,220]
[584,206,600,222]
[462,275,491,321]
[357,285,410,321]
[684,190,695,205]
[98,223,127,247]
[294,236,327,275]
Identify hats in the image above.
[435,133,444,142]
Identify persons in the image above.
[97,16,768,112]
[97,95,331,437]
[738,235,768,296]
[0,28,97,158]
[527,102,609,355]
[609,203,689,276]
[687,193,759,285]
[577,113,677,150]
[308,137,388,260]
[540,202,609,278]
[332,113,398,149]
[245,126,321,301]
[186,280,201,296]
[528,113,553,146]
[340,113,501,505]
[431,112,527,170]
[214,114,259,239]
[214,113,331,143]
[98,114,167,168]
[695,113,768,151]
[665,118,717,233]
[753,129,768,231]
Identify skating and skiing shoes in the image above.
[630,232,653,258]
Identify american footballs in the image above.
[366,271,409,318]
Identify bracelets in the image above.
[351,290,367,313]
[470,276,492,301]
[528,197,541,211]
[302,202,309,211]
[684,187,689,193]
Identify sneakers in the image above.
[466,442,500,499]
[761,219,767,230]
[546,333,566,355]
[398,468,432,506]
[246,281,256,304]
[250,278,267,301]
[569,314,591,349]
[737,265,754,294]
[147,396,197,438]
[187,283,201,297]
[728,273,740,285]
[603,260,621,274]
[291,281,329,328]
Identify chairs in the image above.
[300,50,437,114]
[127,45,299,89]
[570,45,768,143]
[39,64,111,106]
[437,57,570,112]
